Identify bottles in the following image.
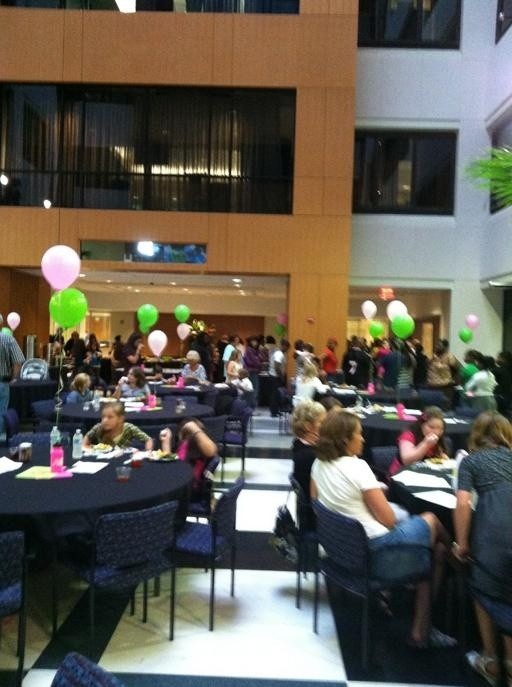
[93,392,101,406]
[72,429,84,457]
[149,391,161,406]
[50,425,60,453]
[367,379,374,393]
[141,363,144,372]
[51,441,64,471]
[397,401,405,419]
[178,374,184,388]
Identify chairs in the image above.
[8,431,71,459]
[169,475,246,631]
[309,498,407,671]
[1,527,31,686]
[289,474,317,609]
[368,446,406,483]
[50,500,182,641]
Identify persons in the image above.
[191,332,290,418]
[180,350,207,381]
[309,410,446,649]
[388,405,448,475]
[66,373,94,404]
[82,403,154,450]
[292,399,327,496]
[450,408,512,687]
[52,328,145,377]
[112,367,150,398]
[0,330,25,413]
[159,417,217,489]
[293,335,512,418]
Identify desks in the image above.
[260,360,512,448]
[0,449,196,609]
[1,360,253,472]
[387,453,512,603]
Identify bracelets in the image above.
[191,430,202,438]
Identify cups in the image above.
[117,466,131,480]
[174,399,186,414]
[10,447,19,460]
[129,455,141,466]
[21,441,33,460]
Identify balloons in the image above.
[273,300,480,344]
[3,245,190,358]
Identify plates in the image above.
[425,458,453,467]
[99,396,144,412]
[151,454,179,461]
[147,380,177,390]
[87,445,113,452]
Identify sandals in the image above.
[465,651,502,684]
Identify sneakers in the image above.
[406,628,457,649]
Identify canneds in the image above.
[176,398,183,415]
[18,441,33,465]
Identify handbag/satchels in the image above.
[269,507,300,569]
